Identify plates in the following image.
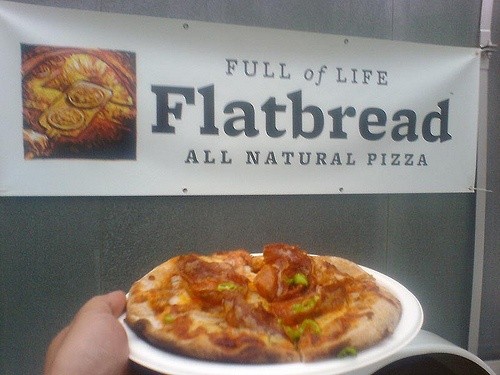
[117,252,424,374]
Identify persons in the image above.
[44,290,130,375]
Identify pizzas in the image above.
[124,242,402,364]
